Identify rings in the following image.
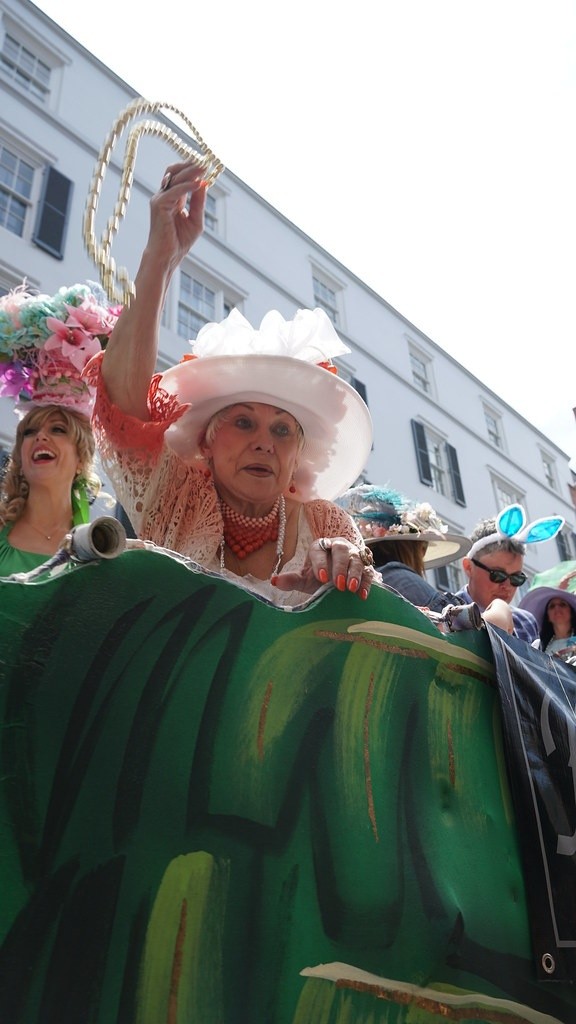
[162,172,171,190]
[350,547,374,566]
[310,537,331,553]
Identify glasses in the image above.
[547,601,567,609]
[471,558,528,586]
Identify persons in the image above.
[518,587,576,668]
[334,485,514,634]
[0,404,101,581]
[456,504,565,651]
[81,160,384,608]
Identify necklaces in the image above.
[21,516,72,540]
[218,494,286,579]
[84,99,225,310]
[545,635,572,652]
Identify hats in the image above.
[334,483,449,545]
[0,280,123,417]
[150,306,374,503]
[517,560,576,634]
